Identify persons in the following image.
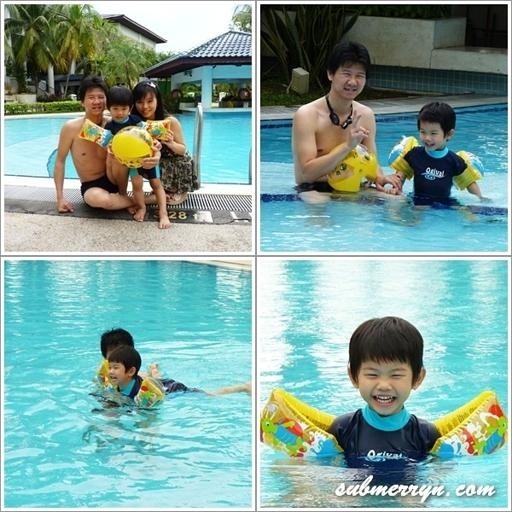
[326,315,444,484]
[54,77,201,231]
[96,326,161,409]
[291,40,403,206]
[106,345,251,409]
[385,101,481,208]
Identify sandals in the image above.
[167,191,187,205]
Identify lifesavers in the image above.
[238,88,251,100]
[260,387,507,464]
[388,135,484,189]
[79,118,171,152]
[97,360,165,407]
[171,89,183,98]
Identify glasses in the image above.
[330,111,352,129]
[134,82,157,89]
